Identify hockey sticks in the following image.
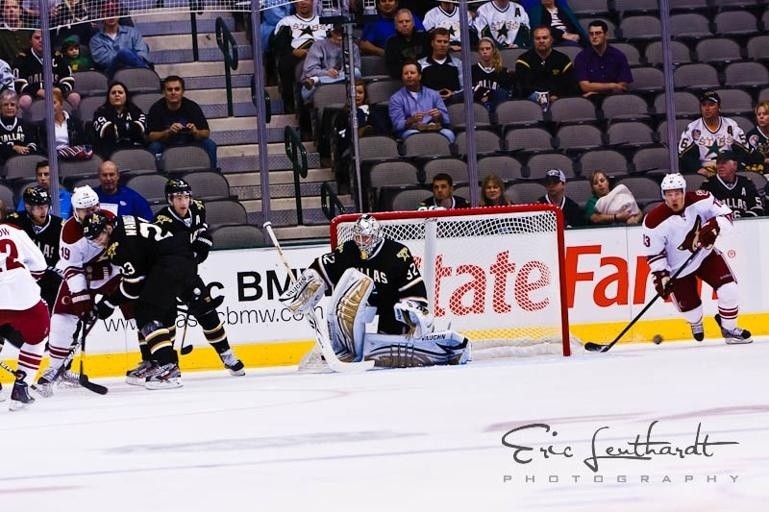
[585,246,702,353]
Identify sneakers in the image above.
[690,316,704,341]
[128,360,181,382]
[11,382,34,403]
[220,349,244,371]
[38,368,79,387]
[721,325,750,339]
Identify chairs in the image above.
[0,0,270,250]
[275,1,768,216]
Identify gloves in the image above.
[701,217,720,250]
[73,291,117,319]
[652,269,673,297]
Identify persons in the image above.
[309,216,427,370]
[642,174,750,340]
[259,0,769,236]
[0,0,244,403]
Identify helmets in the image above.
[661,171,687,202]
[71,182,110,240]
[165,177,193,204]
[23,185,50,205]
[351,212,384,261]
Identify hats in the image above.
[545,168,566,183]
[699,90,720,103]
[711,150,736,161]
